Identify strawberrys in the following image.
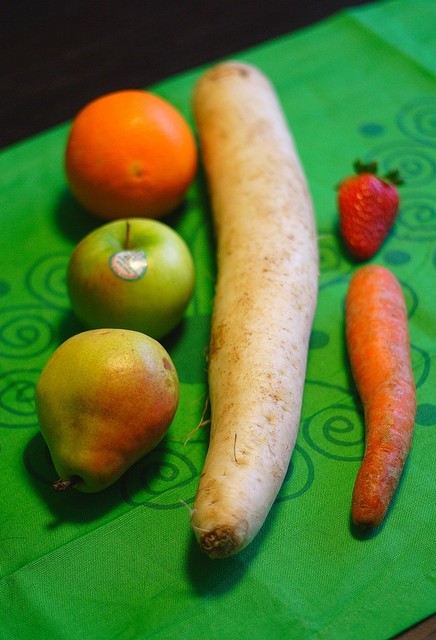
[333,157,404,263]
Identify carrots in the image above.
[342,260,418,532]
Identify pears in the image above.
[32,327,180,498]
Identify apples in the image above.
[66,216,195,342]
